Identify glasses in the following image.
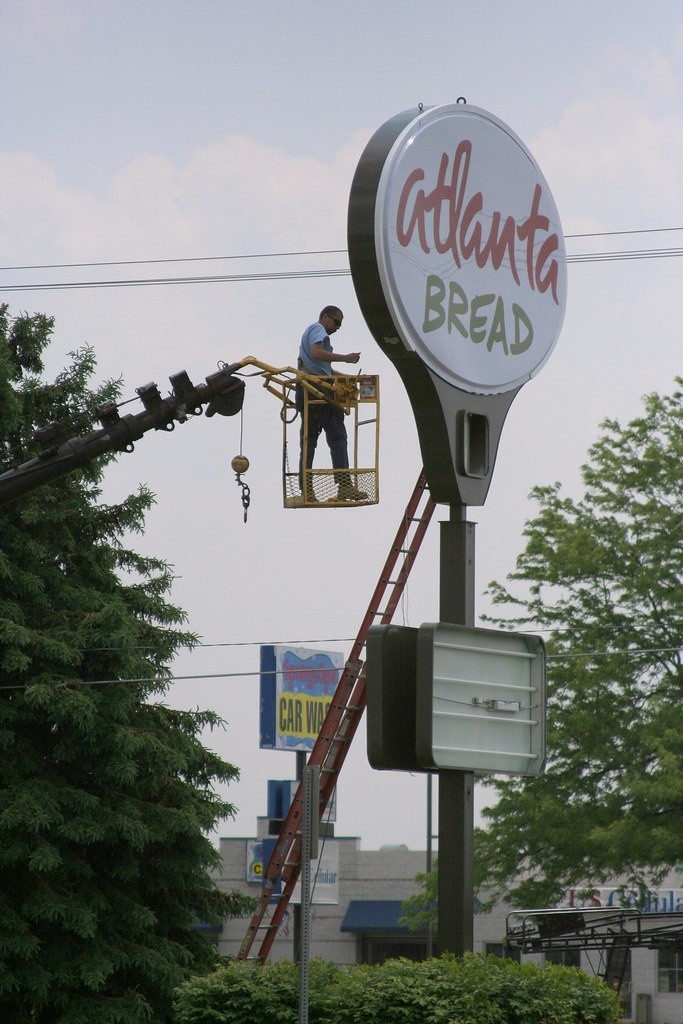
[327,314,341,326]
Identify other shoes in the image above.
[301,493,319,502]
[337,486,368,500]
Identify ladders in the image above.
[234,465,435,967]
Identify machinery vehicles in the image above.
[0,354,383,522]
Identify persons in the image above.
[295,305,367,503]
